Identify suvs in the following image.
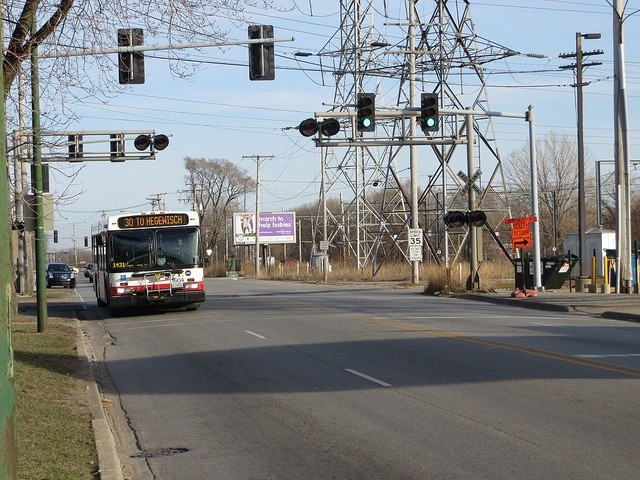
[44,262,76,289]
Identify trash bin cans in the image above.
[515,257,579,290]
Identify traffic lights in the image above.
[321,118,339,136]
[421,92,438,131]
[358,93,375,132]
[300,118,318,136]
[84,236,88,247]
[133,134,169,150]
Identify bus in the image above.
[90,210,205,314]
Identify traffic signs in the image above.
[408,228,423,246]
[504,216,538,248]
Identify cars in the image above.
[88,266,93,282]
[83,263,92,277]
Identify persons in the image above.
[174,238,186,256]
